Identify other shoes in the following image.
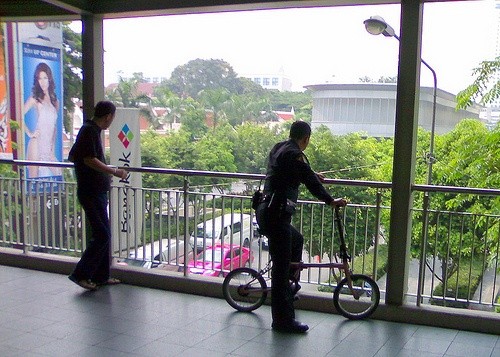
[270,319,309,333]
[97,278,120,285]
[75,279,97,290]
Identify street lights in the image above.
[363,15,452,303]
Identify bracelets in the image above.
[113,168,117,175]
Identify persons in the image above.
[253,120,349,334]
[67,100,129,289]
[24,62,63,177]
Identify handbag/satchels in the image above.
[252,190,263,210]
[280,197,298,212]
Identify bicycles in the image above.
[223,205,380,320]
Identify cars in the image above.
[187,242,254,277]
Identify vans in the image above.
[189,213,269,251]
[122,239,193,268]
